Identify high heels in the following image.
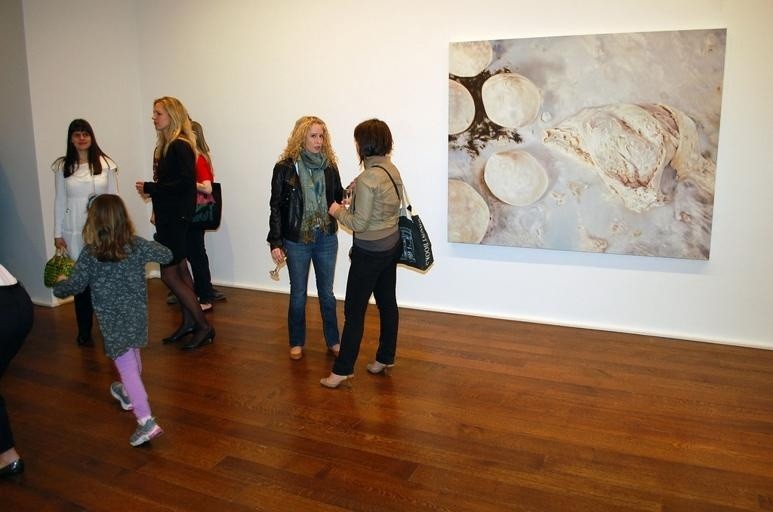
[179,326,216,350]
[320,373,355,388]
[366,361,395,377]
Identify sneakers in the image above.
[109,381,133,411]
[128,419,164,446]
[166,288,226,311]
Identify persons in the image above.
[266,115,356,361]
[135,95,216,349]
[53,192,175,447]
[52,118,123,347]
[163,120,227,303]
[318,117,404,389]
[0,263,33,479]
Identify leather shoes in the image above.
[1,458,24,479]
[328,344,340,357]
[162,324,193,343]
[290,346,302,361]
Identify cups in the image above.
[342,189,351,210]
[136,178,152,206]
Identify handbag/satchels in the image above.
[44,247,76,288]
[397,195,434,274]
[196,183,223,230]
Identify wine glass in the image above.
[268,247,288,282]
